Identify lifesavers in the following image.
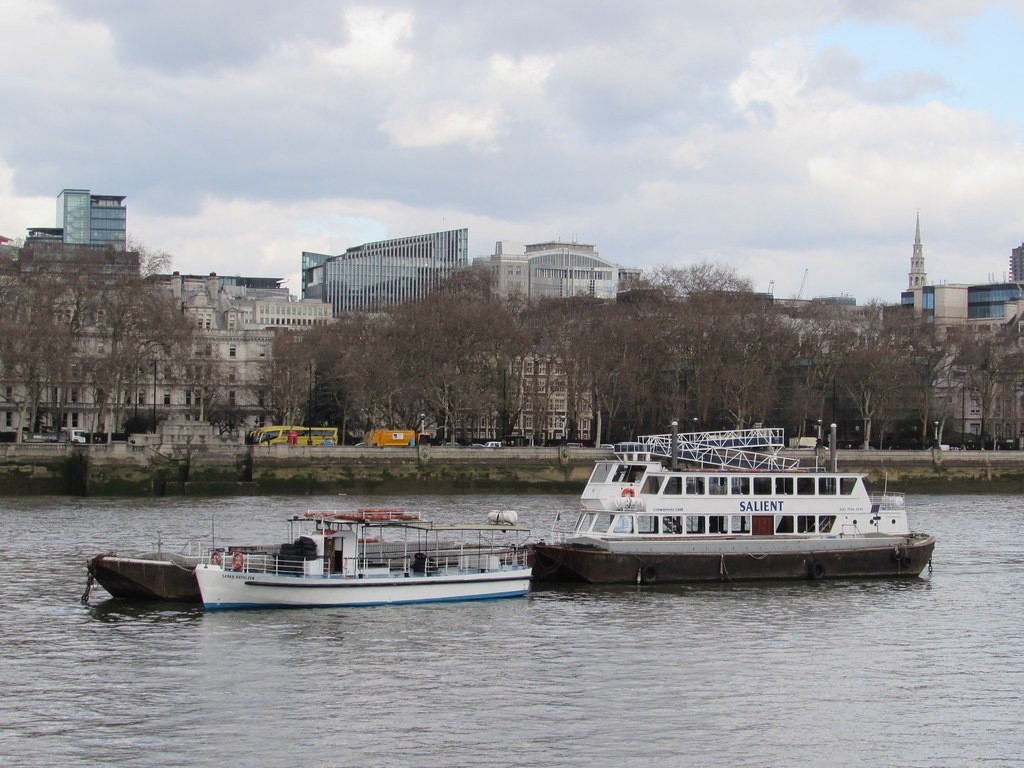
[810,561,825,579]
[641,564,660,583]
[232,552,243,570]
[902,556,913,568]
[280,536,317,556]
[211,551,221,565]
[621,488,636,497]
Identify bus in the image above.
[250,426,338,446]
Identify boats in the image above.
[89,502,532,612]
[532,421,936,583]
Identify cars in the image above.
[442,442,502,448]
[72,430,89,443]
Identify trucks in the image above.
[373,429,431,448]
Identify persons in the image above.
[333,538,343,572]
[324,539,332,572]
